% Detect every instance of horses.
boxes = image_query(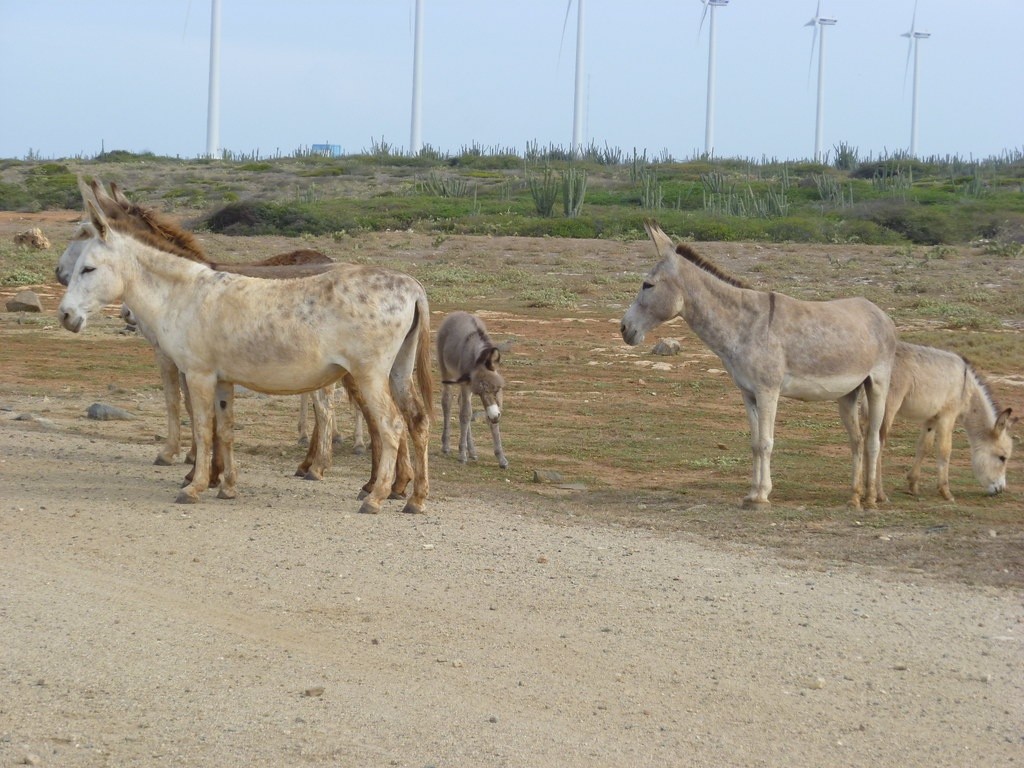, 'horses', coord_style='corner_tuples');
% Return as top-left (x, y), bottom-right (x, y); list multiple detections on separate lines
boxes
(55, 173), (436, 515)
(437, 311), (508, 469)
(619, 218), (1013, 513)
